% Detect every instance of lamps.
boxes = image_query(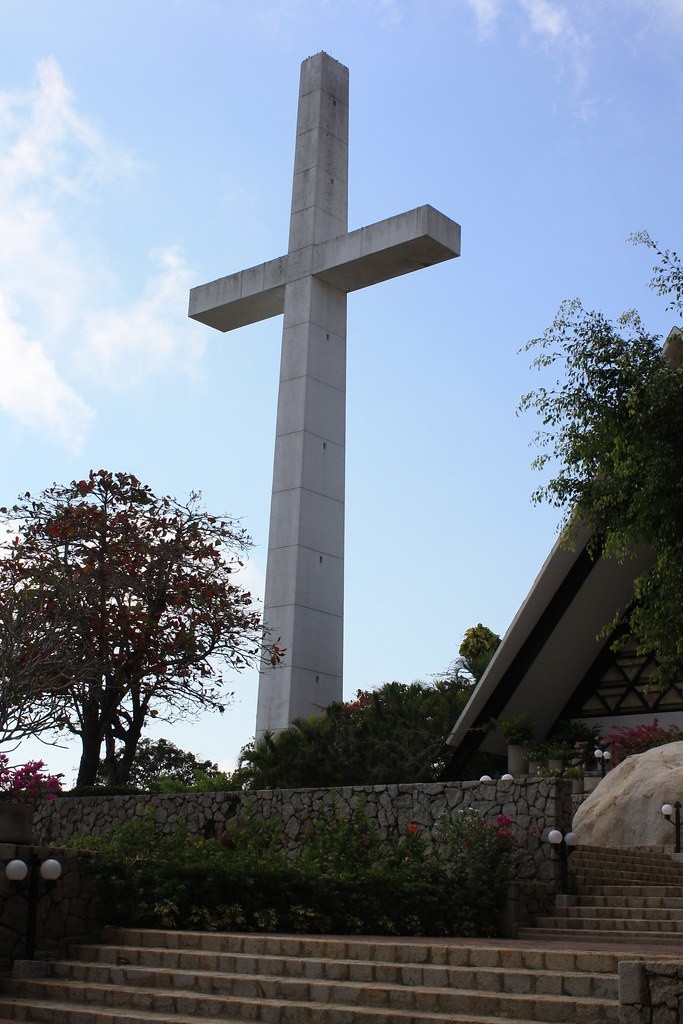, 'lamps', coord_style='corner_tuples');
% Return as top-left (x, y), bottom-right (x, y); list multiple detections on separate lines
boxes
(479, 774), (513, 781)
(4, 857), (62, 897)
(661, 803), (673, 820)
(594, 749), (611, 764)
(547, 827), (578, 858)
